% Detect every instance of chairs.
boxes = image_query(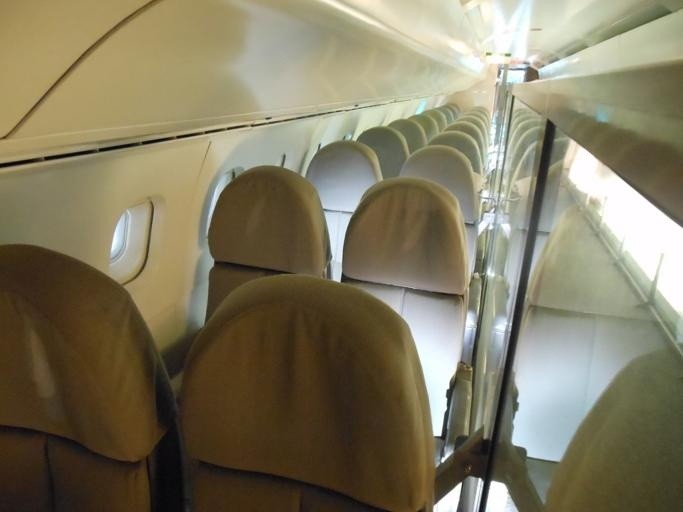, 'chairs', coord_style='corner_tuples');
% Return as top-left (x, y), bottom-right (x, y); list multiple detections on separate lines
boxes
(169, 272), (437, 511)
(0, 102), (485, 368)
(340, 171), (474, 476)
(0, 241), (170, 512)
(488, 96), (683, 512)
(203, 158), (336, 326)
